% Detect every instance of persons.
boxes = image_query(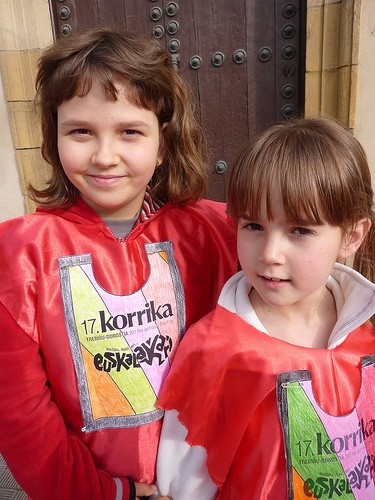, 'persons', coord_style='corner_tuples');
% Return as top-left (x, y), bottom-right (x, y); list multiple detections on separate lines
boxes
(155, 115), (375, 499)
(0, 29), (243, 500)
